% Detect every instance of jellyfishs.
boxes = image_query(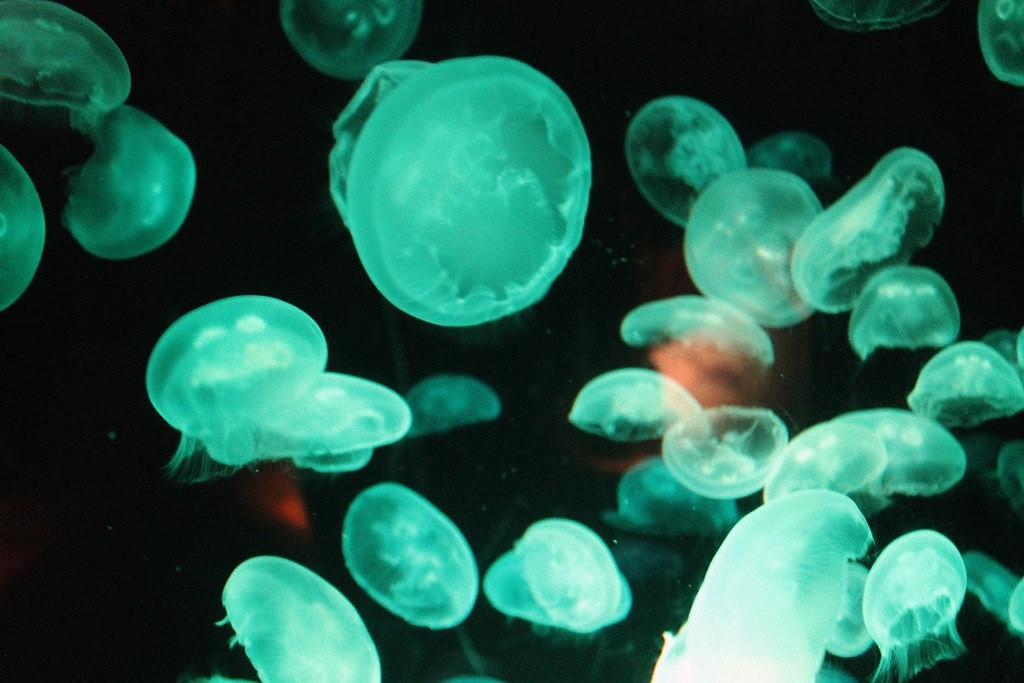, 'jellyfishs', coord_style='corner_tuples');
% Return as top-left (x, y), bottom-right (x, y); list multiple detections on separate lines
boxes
(2, 1), (1023, 681)
